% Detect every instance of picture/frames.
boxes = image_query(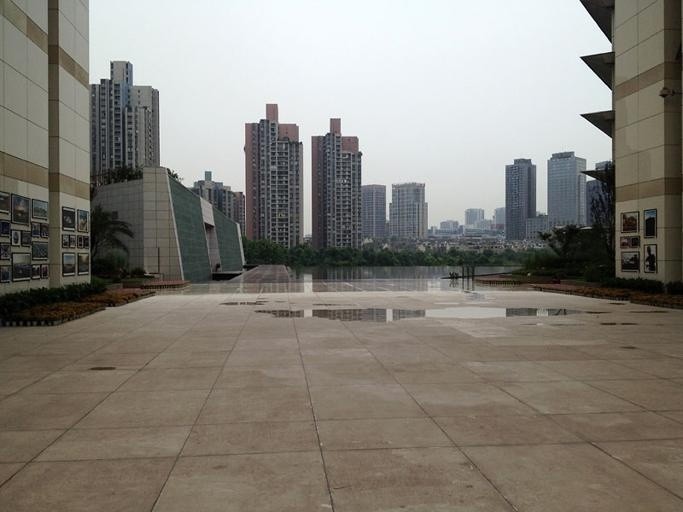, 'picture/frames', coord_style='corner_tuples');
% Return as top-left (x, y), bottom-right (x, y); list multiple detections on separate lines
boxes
(0, 190), (50, 283)
(61, 206), (90, 278)
(619, 208), (658, 273)
